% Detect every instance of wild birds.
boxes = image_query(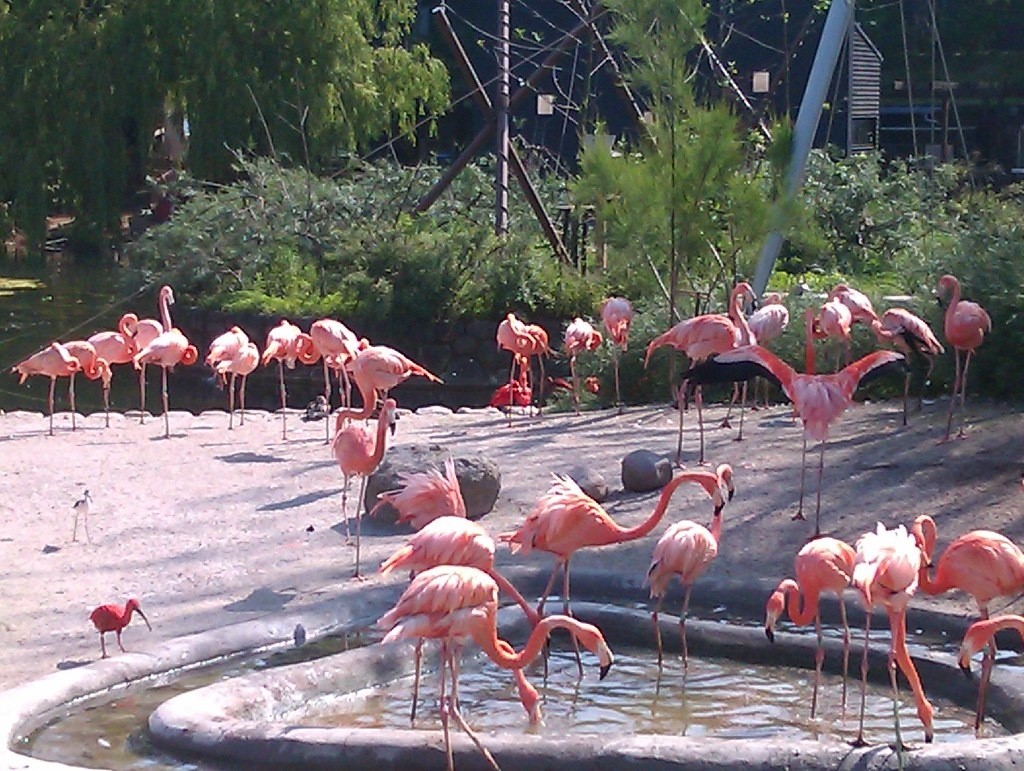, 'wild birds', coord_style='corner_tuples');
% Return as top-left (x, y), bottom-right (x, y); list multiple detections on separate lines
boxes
(12, 273), (1023, 738)
